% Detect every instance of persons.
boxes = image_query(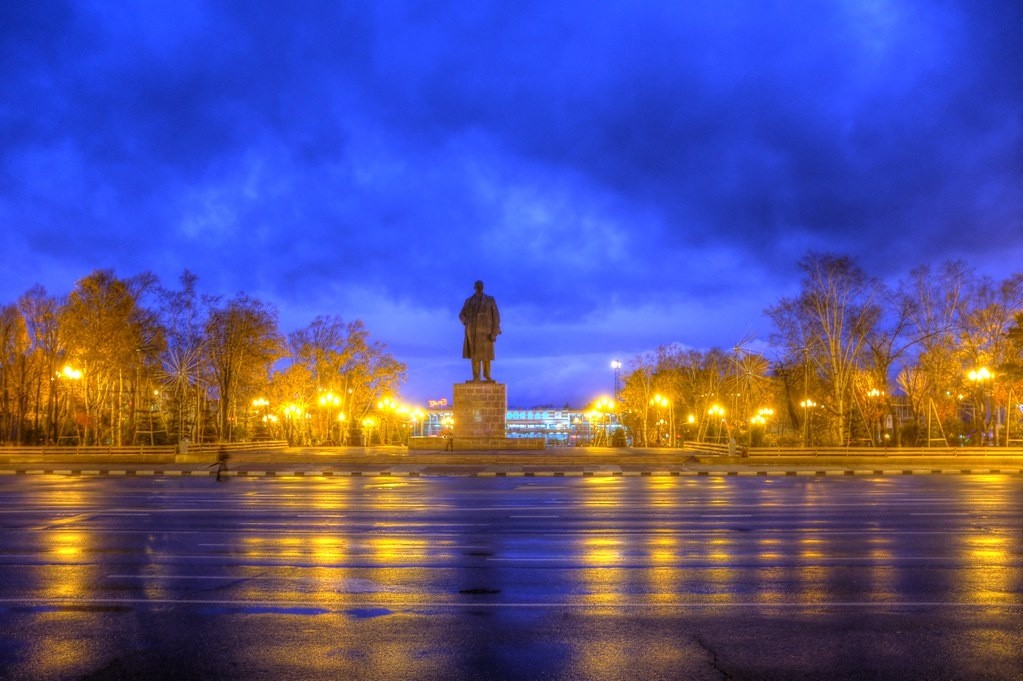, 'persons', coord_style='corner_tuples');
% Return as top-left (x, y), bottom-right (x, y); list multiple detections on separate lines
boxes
(445, 430), (456, 452)
(216, 445), (231, 483)
(458, 280), (502, 383)
